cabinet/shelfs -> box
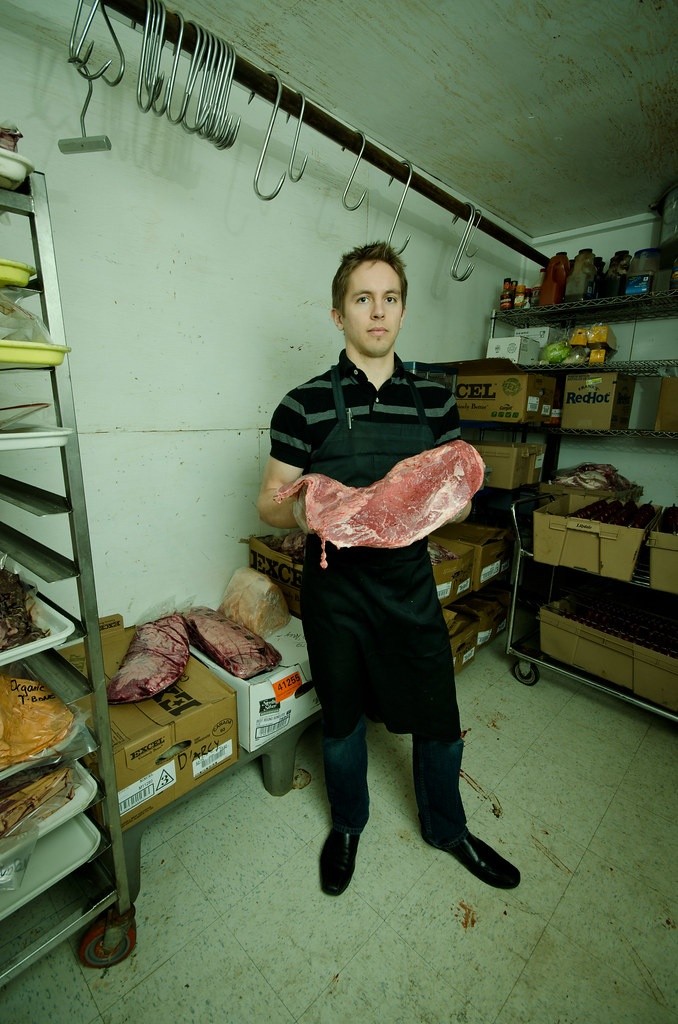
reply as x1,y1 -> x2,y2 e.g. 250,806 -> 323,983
0,170 -> 139,987
491,288 -> 678,439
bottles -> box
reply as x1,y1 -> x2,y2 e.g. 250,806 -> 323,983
500,248 -> 658,310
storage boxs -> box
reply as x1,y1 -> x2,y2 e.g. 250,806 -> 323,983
536,597 -> 633,691
560,371 -> 637,430
427,356 -> 557,423
247,525 -> 515,674
189,617 -> 322,753
514,326 -> 561,349
569,324 -> 618,364
490,440 -> 546,484
469,440 -> 531,490
632,642 -> 678,711
486,336 -> 540,365
655,376 -> 678,432
644,506 -> 678,594
56,614 -> 239,832
532,493 -> 663,582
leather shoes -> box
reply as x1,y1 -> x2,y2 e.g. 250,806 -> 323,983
419,818 -> 521,889
319,827 -> 360,895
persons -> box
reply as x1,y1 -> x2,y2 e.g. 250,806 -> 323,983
257,242 -> 522,896
